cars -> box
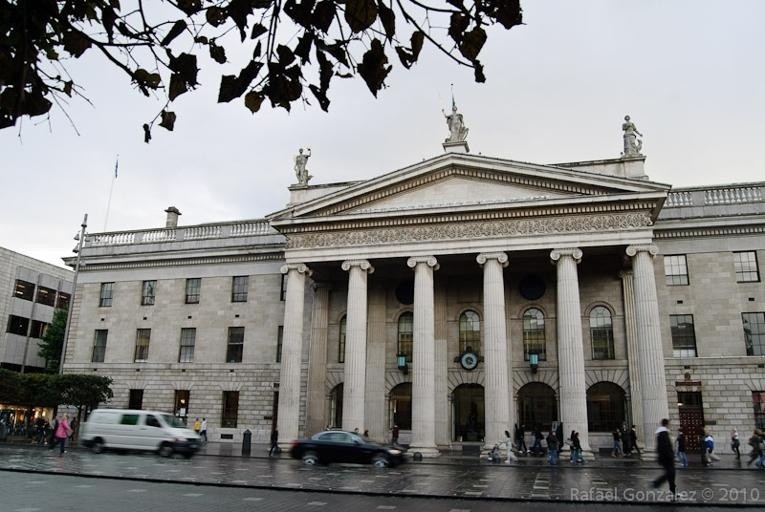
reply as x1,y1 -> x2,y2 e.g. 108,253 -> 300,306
288,429 -> 404,471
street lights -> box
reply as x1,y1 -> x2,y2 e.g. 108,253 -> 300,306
56,213 -> 91,377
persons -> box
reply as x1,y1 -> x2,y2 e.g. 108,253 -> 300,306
676,427 -> 689,465
69,415 -> 76,439
745,429 -> 762,464
390,424 -> 400,442
268,426 -> 279,457
194,415 -> 200,432
324,424 -> 331,430
705,432 -> 721,463
354,427 -> 359,432
699,427 -> 706,463
650,417 -> 681,502
50,411 -> 73,453
50,414 -> 58,430
293,147 -> 311,184
363,429 -> 369,436
199,417 -> 207,441
755,431 -> 765,467
731,426 -> 741,458
622,115 -> 643,150
499,419 -> 643,463
440,106 -> 465,142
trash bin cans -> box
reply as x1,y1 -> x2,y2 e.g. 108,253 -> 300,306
243,429 -> 252,445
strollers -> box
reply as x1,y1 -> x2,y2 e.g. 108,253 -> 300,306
487,442 -> 503,462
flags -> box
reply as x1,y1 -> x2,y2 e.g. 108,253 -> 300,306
114,161 -> 118,178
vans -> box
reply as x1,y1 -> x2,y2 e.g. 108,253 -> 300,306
82,407 -> 203,459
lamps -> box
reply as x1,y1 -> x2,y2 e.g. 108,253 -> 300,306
396,351 -> 408,374
528,349 -> 539,373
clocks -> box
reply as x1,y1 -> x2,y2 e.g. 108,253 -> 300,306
460,351 -> 478,369
